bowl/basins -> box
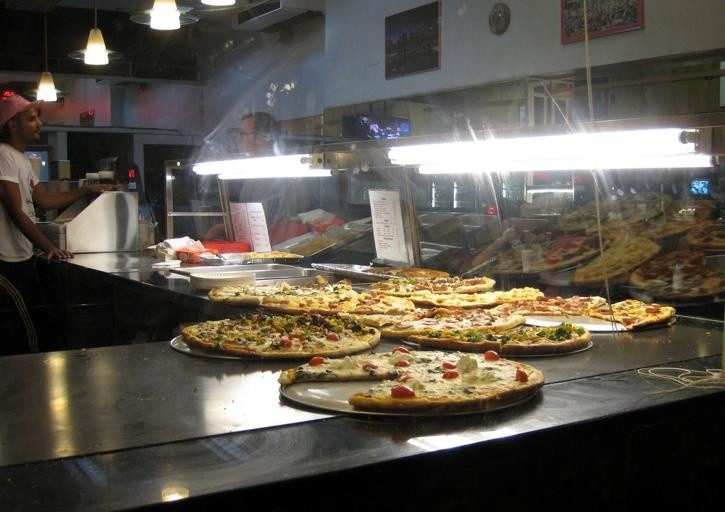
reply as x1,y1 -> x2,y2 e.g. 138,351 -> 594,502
189,271 -> 252,292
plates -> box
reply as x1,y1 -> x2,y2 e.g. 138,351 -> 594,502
168,335 -> 256,363
250,271 -> 335,287
276,371 -> 542,419
398,336 -> 596,359
630,253 -> 725,308
311,263 -> 449,282
171,263 -> 302,272
523,314 -> 634,335
543,260 -> 585,277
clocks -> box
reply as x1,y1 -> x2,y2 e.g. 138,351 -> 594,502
488,3 -> 511,35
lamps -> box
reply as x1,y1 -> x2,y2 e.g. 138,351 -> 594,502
191,155 -> 312,176
412,152 -> 725,175
217,167 -> 338,180
84,28 -> 111,66
149,0 -> 182,31
384,126 -> 703,167
36,71 -> 58,103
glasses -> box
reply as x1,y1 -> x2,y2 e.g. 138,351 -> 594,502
236,132 -> 253,140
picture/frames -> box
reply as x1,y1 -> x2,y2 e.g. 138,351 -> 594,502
559,0 -> 645,46
384,1 -> 442,81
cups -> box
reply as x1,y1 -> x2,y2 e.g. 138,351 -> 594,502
522,248 -> 534,276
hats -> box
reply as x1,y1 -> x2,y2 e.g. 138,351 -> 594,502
0,94 -> 45,127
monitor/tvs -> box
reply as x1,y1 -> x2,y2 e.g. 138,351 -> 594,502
357,115 -> 410,139
21,145 -> 50,180
688,180 -> 709,196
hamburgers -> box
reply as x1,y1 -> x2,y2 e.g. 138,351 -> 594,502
639,199 -> 712,237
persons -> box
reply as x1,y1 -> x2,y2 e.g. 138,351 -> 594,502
2,92 -> 75,297
199,108 -> 297,242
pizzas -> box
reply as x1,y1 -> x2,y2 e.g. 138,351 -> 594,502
403,286 -> 545,308
257,290 -> 415,315
473,228 -> 610,277
688,218 -> 725,247
519,294 -> 608,316
407,325 -> 590,354
277,347 -> 544,413
182,314 -> 383,355
364,266 -> 449,279
367,276 -> 496,296
572,234 -> 662,284
591,297 -> 677,329
559,189 -> 672,227
630,249 -> 725,300
382,304 -> 527,337
207,275 -> 353,306
